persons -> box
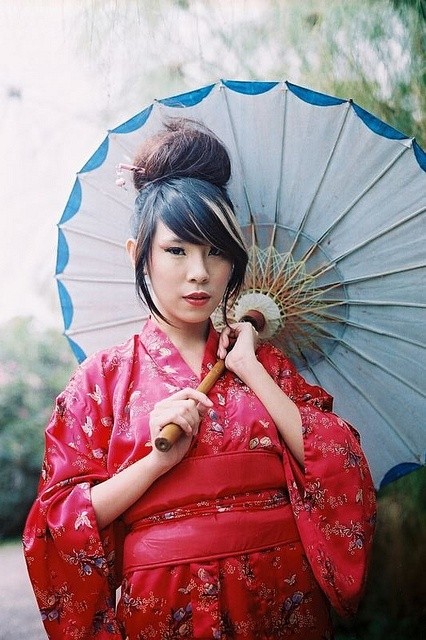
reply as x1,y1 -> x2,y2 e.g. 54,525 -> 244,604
22,116 -> 378,640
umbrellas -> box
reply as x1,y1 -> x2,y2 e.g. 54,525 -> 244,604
53,79 -> 426,492
